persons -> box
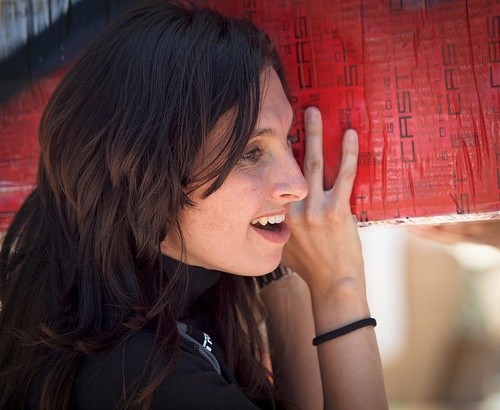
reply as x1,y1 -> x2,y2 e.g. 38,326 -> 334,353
0,0 -> 389,410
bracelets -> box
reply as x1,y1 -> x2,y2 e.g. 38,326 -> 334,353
255,264 -> 296,289
312,318 -> 376,346
